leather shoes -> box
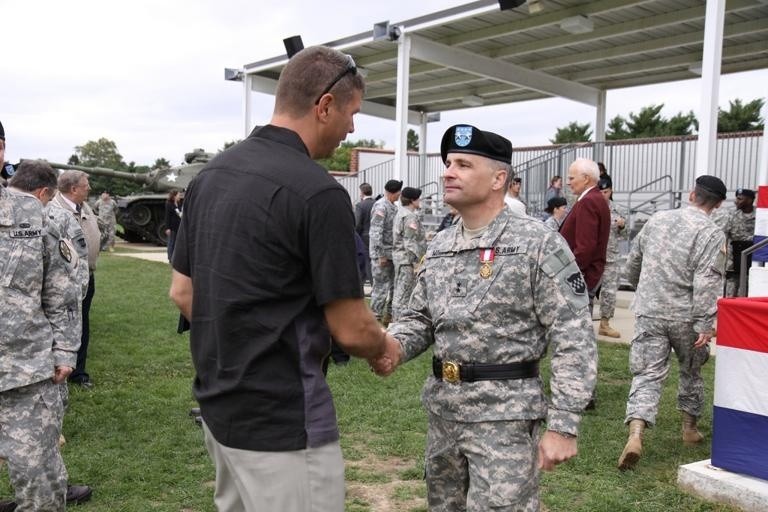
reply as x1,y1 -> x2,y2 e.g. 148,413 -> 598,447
65,485 -> 92,503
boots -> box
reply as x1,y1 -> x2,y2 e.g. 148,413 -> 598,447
680,409 -> 705,443
595,316 -> 624,339
617,415 -> 647,472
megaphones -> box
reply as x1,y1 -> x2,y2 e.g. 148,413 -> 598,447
427,112 -> 440,122
225,68 -> 243,81
374,21 -> 392,42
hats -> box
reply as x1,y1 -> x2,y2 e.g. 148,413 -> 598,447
384,179 -> 403,193
599,178 -> 611,190
735,189 -> 755,198
441,124 -> 513,164
547,197 -> 567,208
695,175 -> 726,198
401,187 -> 422,199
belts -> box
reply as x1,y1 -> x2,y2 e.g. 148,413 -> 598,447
429,355 -> 541,384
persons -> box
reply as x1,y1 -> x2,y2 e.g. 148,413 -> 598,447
164,187 -> 187,262
1,159 -> 101,512
93,190 -> 120,252
370,124 -> 597,512
1,122 -> 67,512
708,189 -> 757,337
353,162 -> 626,338
168,45 -> 403,511
618,175 -> 728,471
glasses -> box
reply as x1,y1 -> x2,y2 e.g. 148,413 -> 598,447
314,49 -> 359,108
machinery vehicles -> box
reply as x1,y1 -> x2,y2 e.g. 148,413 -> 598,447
19,148 -> 222,247
